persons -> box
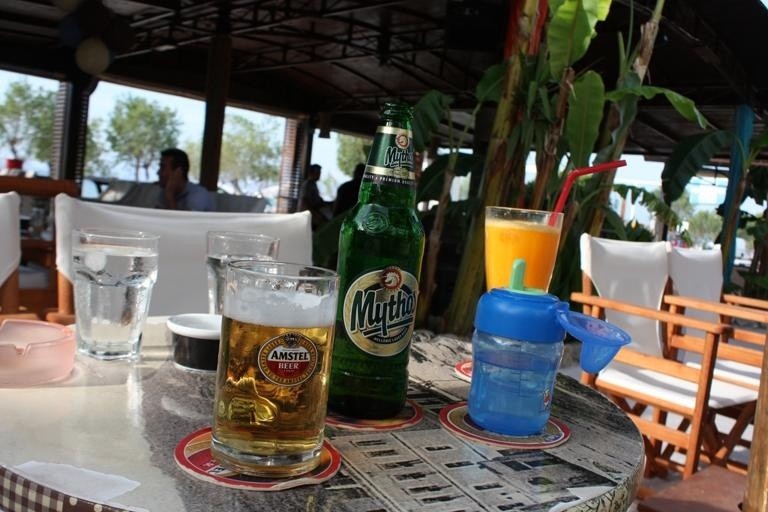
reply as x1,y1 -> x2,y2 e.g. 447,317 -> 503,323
143,149 -> 215,212
295,164 -> 331,230
332,162 -> 366,216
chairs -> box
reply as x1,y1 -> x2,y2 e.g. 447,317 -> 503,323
2,187 -> 27,316
654,238 -> 767,479
568,229 -> 767,484
113,181 -> 267,213
48,194 -> 322,319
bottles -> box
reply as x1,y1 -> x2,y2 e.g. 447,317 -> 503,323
470,282 -> 632,436
327,96 -> 427,420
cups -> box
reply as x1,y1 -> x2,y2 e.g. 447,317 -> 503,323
205,229 -> 282,316
71,226 -> 163,360
212,259 -> 339,479
483,203 -> 563,291
20,216 -> 31,230
168,313 -> 224,377
31,200 -> 48,228
160,382 -> 213,417
